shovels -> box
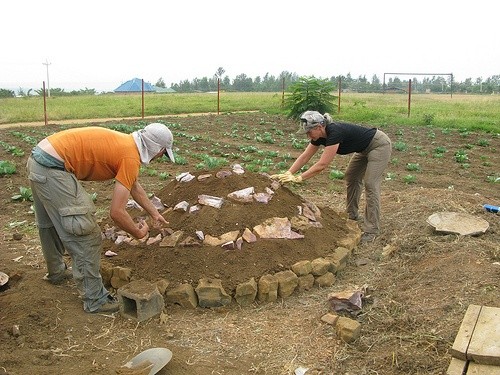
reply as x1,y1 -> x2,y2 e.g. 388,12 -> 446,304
116,347 -> 173,375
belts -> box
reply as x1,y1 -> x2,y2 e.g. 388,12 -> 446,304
29,152 -> 68,171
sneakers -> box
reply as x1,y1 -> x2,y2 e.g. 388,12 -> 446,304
93,294 -> 121,313
51,263 -> 73,283
361,232 -> 376,242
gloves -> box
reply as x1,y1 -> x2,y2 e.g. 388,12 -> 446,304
269,171 -> 303,185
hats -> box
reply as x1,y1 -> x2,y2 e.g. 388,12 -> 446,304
295,110 -> 324,135
131,123 -> 175,165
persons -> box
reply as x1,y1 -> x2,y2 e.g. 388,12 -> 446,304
269,110 -> 392,243
25,122 -> 176,313
